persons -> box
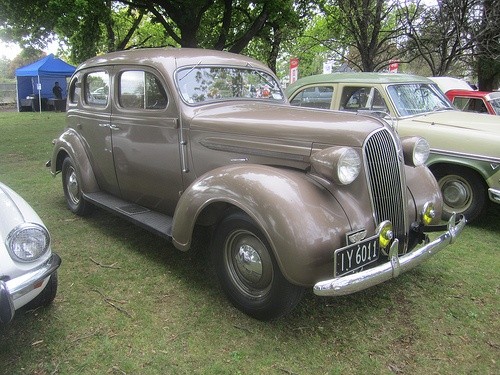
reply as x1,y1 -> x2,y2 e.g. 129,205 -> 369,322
53,82 -> 63,99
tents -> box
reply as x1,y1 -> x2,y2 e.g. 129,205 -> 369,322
15,53 -> 77,113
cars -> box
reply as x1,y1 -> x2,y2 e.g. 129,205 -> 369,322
0,181 -> 62,328
47,46 -> 467,322
445,89 -> 500,116
286,73 -> 500,225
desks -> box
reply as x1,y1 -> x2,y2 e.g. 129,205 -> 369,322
26,98 -> 38,112
43,97 -> 61,113
